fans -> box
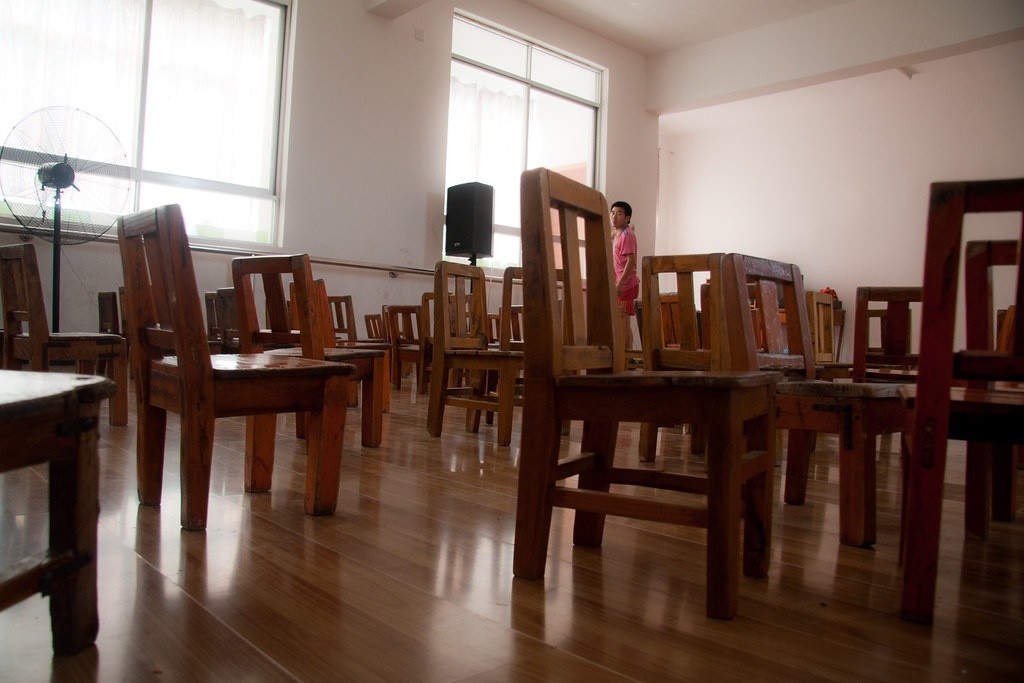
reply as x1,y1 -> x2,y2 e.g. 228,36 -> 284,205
0,105 -> 131,334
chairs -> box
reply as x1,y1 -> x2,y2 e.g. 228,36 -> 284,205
0,166 -> 1024,683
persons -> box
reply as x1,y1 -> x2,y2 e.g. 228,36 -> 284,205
610,201 -> 641,372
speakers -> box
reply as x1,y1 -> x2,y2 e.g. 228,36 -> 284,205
446,182 -> 493,260
